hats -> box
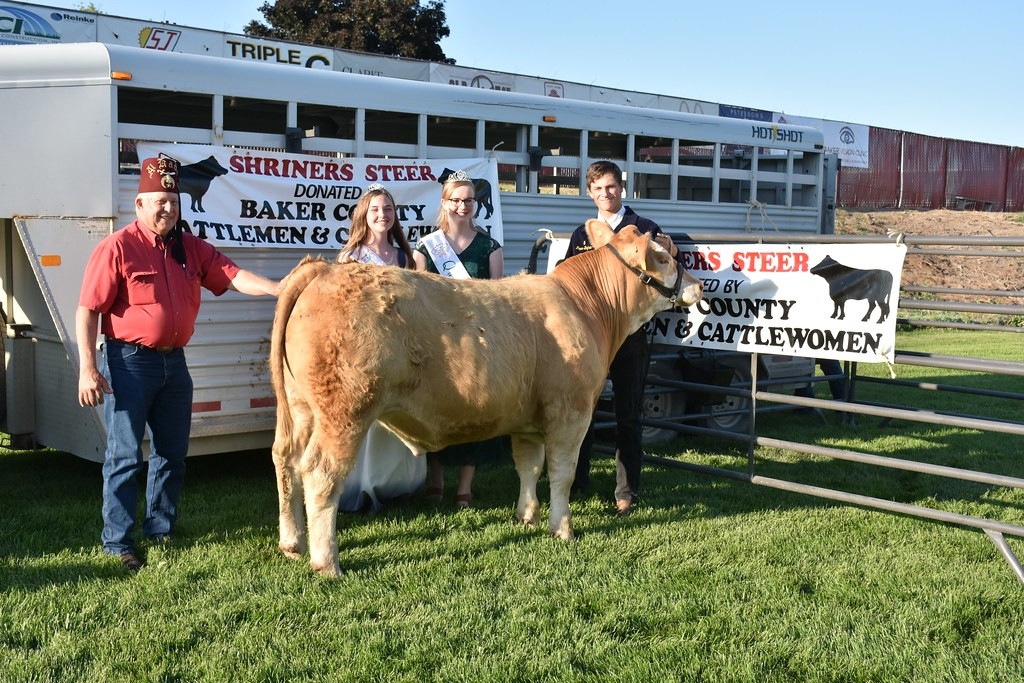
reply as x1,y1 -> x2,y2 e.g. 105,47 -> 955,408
137,158 -> 180,196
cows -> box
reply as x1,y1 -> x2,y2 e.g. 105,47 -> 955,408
267,219 -> 706,579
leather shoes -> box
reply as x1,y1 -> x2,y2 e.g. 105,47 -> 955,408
569,479 -> 591,497
149,533 -> 173,541
116,553 -> 141,569
616,498 -> 632,516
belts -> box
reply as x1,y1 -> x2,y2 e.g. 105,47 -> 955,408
103,332 -> 178,354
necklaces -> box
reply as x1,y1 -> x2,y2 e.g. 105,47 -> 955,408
384,254 -> 394,265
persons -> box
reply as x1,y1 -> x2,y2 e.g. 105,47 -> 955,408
337,184 -> 427,517
792,358 -> 857,423
556,160 -> 687,515
409,169 -> 503,512
75,158 -> 291,572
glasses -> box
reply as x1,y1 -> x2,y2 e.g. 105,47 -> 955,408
446,197 -> 477,207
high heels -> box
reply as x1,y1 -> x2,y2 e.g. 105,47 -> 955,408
429,479 -> 445,501
457,484 -> 473,508
